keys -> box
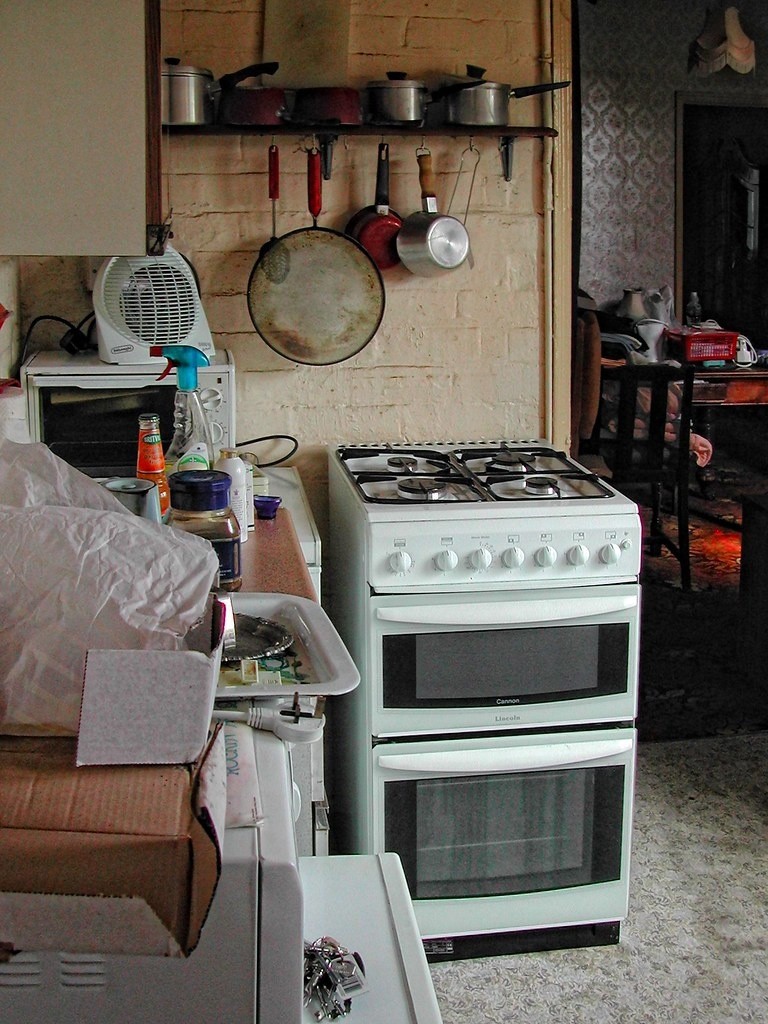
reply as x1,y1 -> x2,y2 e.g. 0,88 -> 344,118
304,936 -> 372,1022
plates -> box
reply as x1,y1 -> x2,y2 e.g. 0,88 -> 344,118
221,614 -> 294,661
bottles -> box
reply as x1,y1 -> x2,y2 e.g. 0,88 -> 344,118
686,292 -> 701,327
163,471 -> 243,593
136,414 -> 170,524
212,448 -> 248,543
238,454 -> 255,531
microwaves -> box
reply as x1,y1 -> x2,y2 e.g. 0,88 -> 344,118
21,350 -> 235,478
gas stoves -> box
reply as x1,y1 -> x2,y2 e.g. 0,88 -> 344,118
328,438 -> 642,593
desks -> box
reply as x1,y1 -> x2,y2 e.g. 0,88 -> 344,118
675,351 -> 768,501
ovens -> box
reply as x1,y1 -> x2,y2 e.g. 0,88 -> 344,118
355,586 -> 642,964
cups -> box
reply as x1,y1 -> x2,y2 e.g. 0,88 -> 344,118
255,495 -> 282,519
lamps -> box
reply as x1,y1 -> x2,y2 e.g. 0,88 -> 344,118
687,6 -> 756,80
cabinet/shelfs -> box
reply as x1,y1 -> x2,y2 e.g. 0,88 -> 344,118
0,0 -> 165,256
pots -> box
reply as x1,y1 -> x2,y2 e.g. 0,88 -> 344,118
397,147 -> 469,277
367,72 -> 487,121
344,143 -> 405,270
160,57 -> 279,127
444,64 -> 571,126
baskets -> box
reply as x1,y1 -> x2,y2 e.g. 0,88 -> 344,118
664,328 -> 740,361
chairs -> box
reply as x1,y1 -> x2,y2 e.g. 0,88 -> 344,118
573,363 -> 696,589
